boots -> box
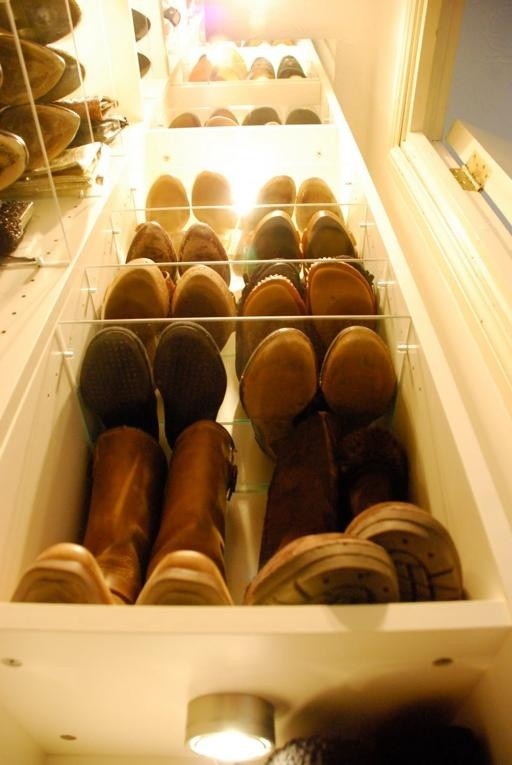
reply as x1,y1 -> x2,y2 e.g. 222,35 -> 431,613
10,410 -> 460,605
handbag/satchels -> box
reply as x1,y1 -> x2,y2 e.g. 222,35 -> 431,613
0,200 -> 34,256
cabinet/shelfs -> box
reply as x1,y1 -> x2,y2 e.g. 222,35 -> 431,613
0,0 -> 512,765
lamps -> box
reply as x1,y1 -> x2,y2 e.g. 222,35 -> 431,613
185,692 -> 275,763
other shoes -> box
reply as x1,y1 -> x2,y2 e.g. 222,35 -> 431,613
81,172 -> 397,452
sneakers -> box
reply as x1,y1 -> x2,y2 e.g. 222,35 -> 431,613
172,49 -> 320,127
0,0 -> 126,191
